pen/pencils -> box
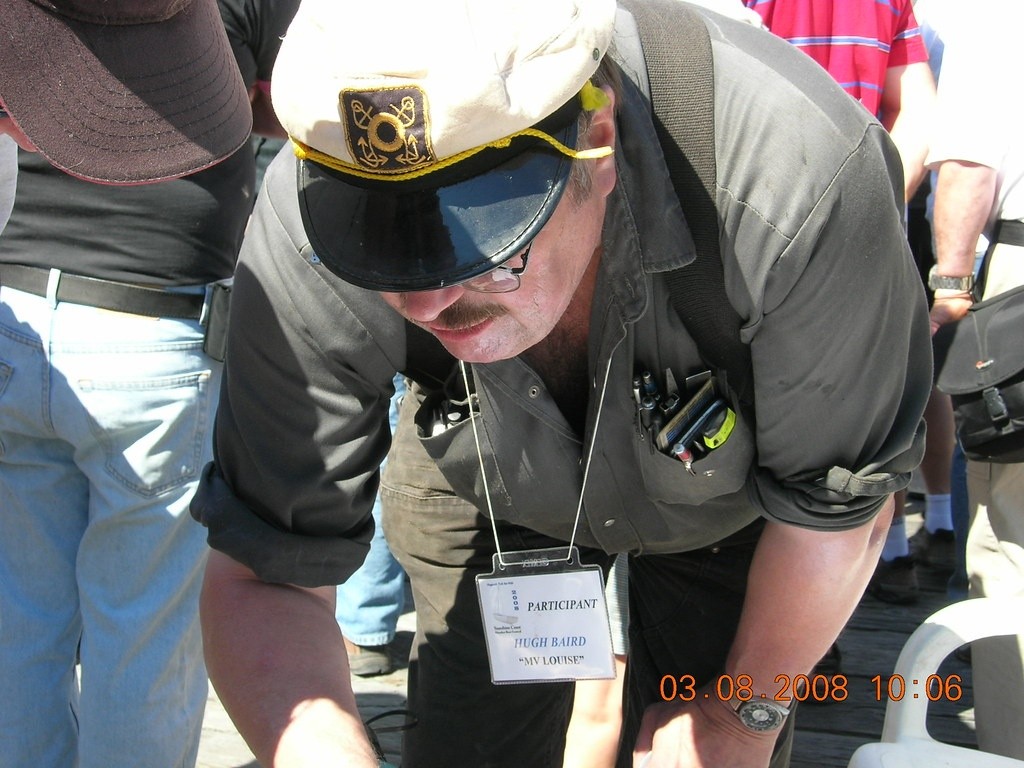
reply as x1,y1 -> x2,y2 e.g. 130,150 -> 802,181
671,443 -> 698,478
633,371 -> 664,455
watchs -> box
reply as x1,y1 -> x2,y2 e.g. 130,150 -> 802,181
714,674 -> 790,731
928,264 -> 975,291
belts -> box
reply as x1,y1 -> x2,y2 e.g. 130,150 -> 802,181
0,262 -> 205,320
992,220 -> 1024,247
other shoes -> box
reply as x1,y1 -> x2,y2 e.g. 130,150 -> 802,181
345,635 -> 392,675
907,526 -> 957,593
868,554 -> 920,602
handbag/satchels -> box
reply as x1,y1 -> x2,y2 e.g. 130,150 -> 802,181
933,285 -> 1024,464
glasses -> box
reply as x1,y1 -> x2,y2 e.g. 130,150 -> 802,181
455,239 -> 532,294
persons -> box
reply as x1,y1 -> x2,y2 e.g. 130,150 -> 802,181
190,0 -> 934,768
0,1 -> 1024,768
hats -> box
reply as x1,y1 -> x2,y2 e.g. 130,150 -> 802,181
0,0 -> 253,185
270,0 -> 609,293
